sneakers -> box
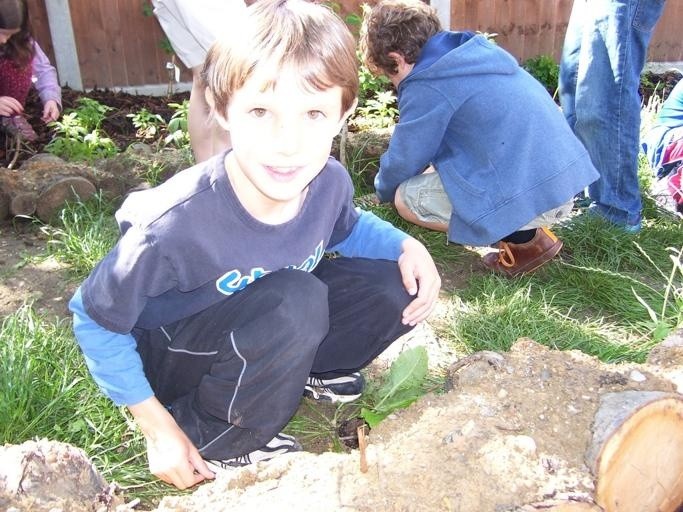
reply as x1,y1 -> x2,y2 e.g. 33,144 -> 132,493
478,225 -> 565,280
302,371 -> 367,404
192,431 -> 304,479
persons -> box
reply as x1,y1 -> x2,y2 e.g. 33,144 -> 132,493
67,0 -> 444,495
1,0 -> 64,143
641,76 -> 682,210
558,0 -> 667,235
151,0 -> 249,166
358,0 -> 600,278
